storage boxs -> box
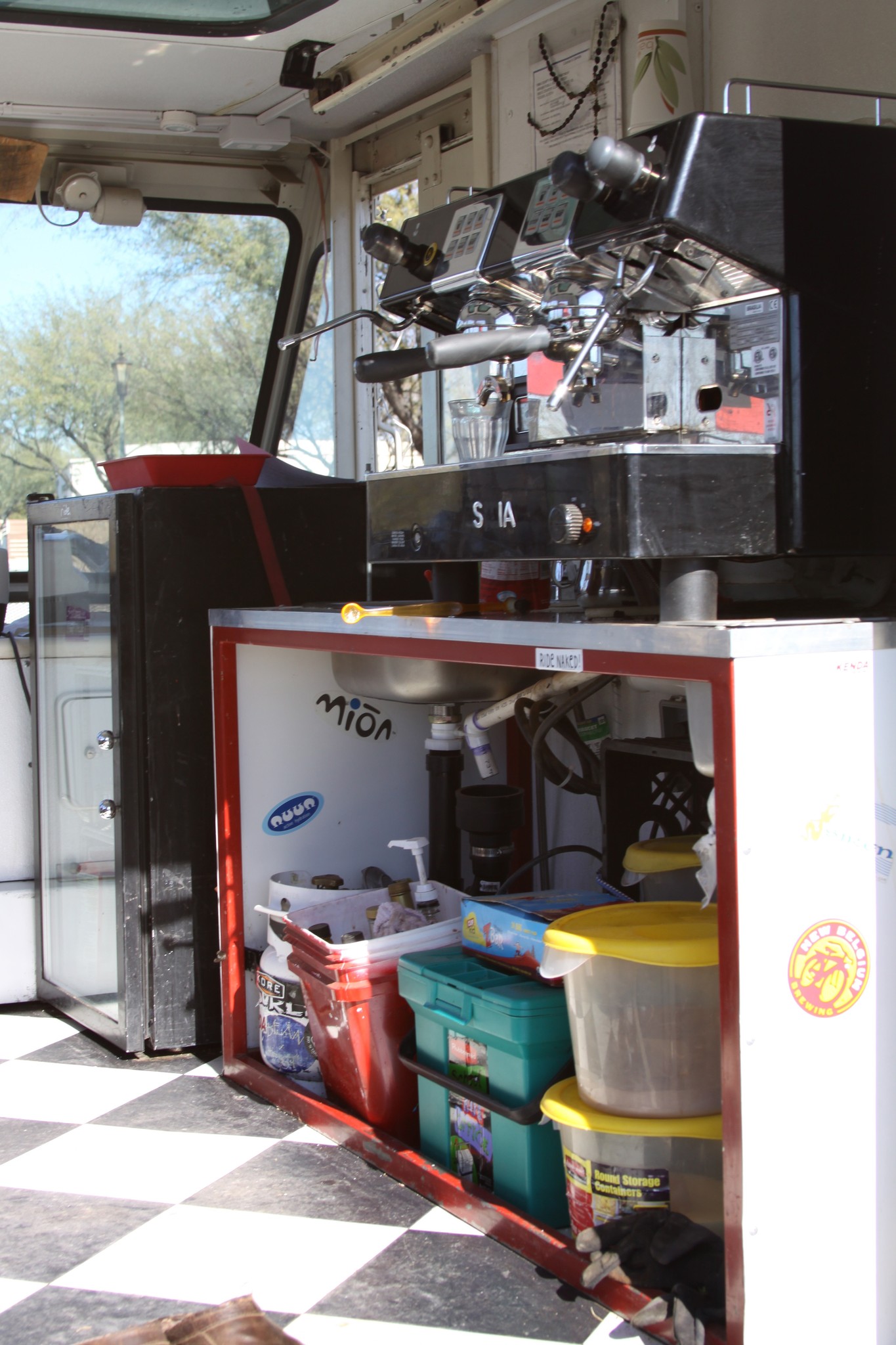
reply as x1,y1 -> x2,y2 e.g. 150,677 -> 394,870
282,737 -> 717,1228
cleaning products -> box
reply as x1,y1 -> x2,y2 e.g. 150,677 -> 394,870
388,836 -> 443,921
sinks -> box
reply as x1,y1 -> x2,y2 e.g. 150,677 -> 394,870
330,605 -> 528,706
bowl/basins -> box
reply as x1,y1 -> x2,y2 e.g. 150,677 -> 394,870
537,1076 -> 726,1242
622,834 -> 707,904
536,902 -> 724,1119
97,454 -> 273,490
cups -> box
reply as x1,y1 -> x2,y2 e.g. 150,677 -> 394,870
448,397 -> 513,462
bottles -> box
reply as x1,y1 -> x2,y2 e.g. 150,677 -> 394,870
309,881 -> 416,943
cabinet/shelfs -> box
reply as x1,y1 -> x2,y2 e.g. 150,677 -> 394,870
211,605 -> 896,1345
